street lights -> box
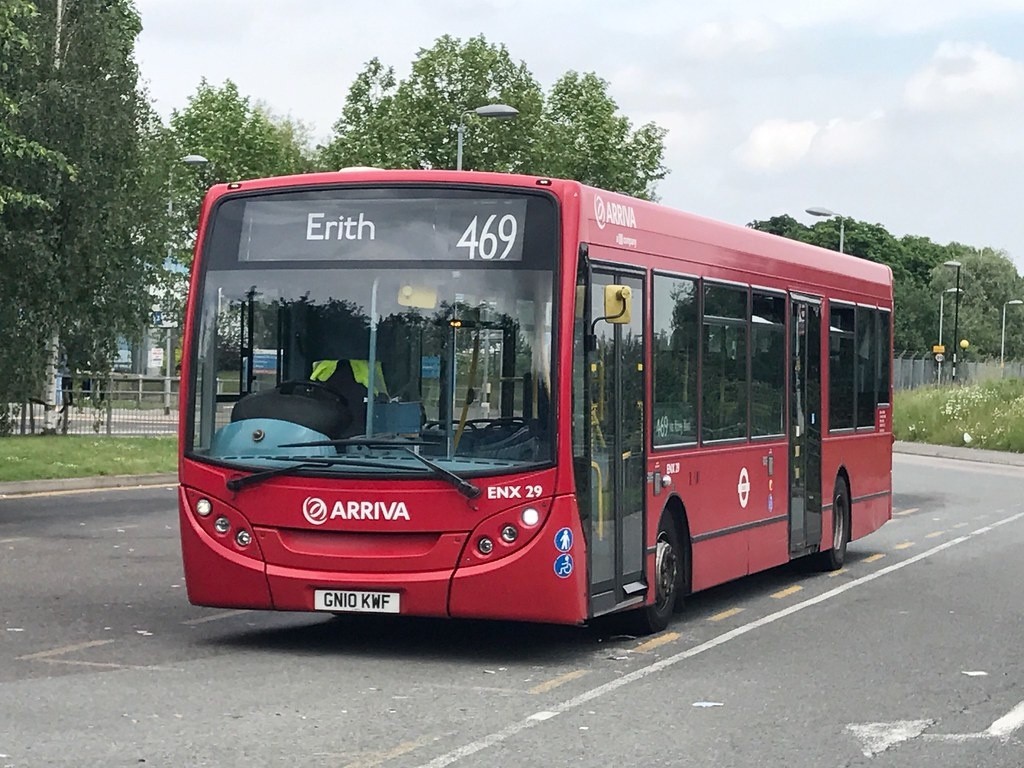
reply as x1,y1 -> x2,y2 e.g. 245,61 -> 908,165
456,104 -> 520,172
943,260 -> 957,386
936,287 -> 963,385
960,340 -> 969,386
805,206 -> 845,253
1000,300 -> 1023,389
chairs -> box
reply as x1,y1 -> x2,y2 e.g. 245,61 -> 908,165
312,357 -> 385,437
428,418 -> 538,458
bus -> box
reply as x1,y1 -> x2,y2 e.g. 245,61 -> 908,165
178,166 -> 895,634
